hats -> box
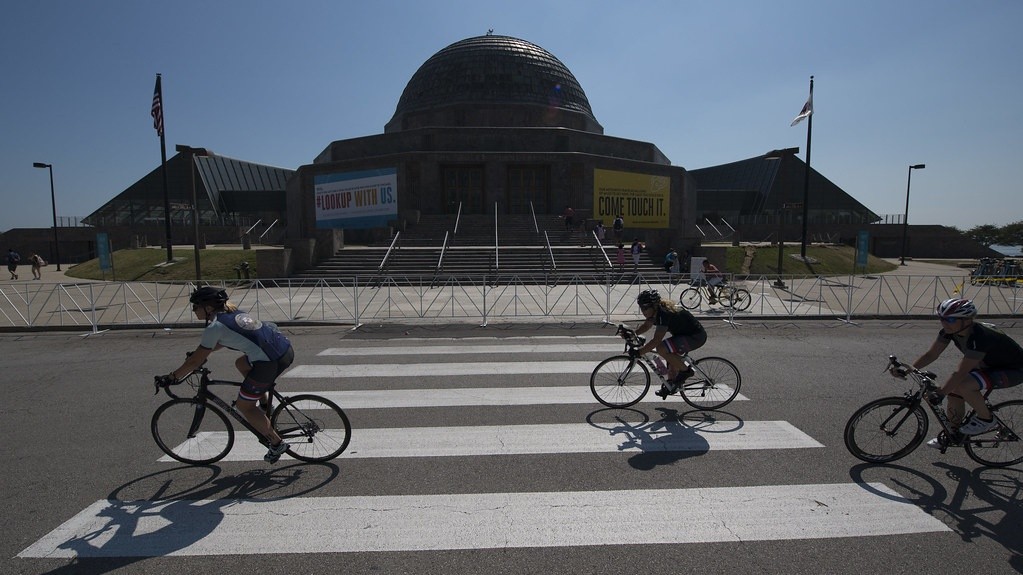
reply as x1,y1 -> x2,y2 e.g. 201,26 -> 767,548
673,252 -> 677,256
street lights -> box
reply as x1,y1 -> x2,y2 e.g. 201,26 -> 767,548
33,162 -> 63,271
764,146 -> 799,286
901,164 -> 925,264
175,143 -> 216,290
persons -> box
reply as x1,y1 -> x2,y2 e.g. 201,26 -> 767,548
27,249 -> 42,281
613,214 -> 625,245
700,260 -> 723,304
630,237 -> 643,268
5,248 -> 20,280
618,290 -> 707,398
888,298 -> 1023,448
563,204 -> 576,231
616,245 -> 626,270
664,247 -> 693,284
596,222 -> 606,246
154,287 -> 294,462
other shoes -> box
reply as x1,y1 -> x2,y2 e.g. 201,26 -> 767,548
655,382 -> 677,395
673,367 -> 694,384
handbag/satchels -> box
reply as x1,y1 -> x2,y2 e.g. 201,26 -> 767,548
691,278 -> 707,287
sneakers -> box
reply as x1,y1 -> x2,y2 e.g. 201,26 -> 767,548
959,415 -> 998,434
927,434 -> 950,448
264,441 -> 290,461
256,404 -> 275,415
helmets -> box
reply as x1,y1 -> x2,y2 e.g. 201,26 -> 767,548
190,287 -> 229,303
936,299 -> 976,317
638,289 -> 661,306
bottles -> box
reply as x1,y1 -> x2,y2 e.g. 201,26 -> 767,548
652,354 -> 667,375
231,400 -> 248,422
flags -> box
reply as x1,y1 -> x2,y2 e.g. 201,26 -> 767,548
151,79 -> 162,137
790,84 -> 815,126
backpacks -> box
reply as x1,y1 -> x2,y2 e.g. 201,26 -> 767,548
614,218 -> 621,230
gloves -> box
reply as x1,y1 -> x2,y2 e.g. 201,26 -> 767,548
929,389 -> 944,405
154,372 -> 178,388
890,367 -> 907,376
628,347 -> 640,356
621,330 -> 634,338
185,351 -> 196,360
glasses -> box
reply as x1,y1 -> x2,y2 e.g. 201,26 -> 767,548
939,317 -> 967,322
193,304 -> 206,310
641,306 -> 653,312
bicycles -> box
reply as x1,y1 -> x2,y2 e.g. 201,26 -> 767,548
589,320 -> 742,411
151,350 -> 352,465
970,256 -> 1014,287
843,354 -> 1023,468
680,269 -> 752,312
